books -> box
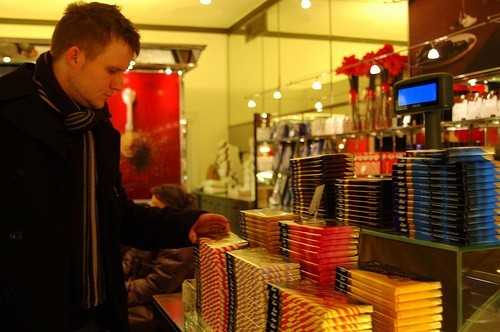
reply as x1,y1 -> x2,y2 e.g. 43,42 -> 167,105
193,207 -> 444,332
290,147 -> 500,247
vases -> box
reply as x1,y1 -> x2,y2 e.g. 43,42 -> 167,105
349,90 -> 390,130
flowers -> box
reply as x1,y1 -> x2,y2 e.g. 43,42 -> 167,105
335,43 -> 408,86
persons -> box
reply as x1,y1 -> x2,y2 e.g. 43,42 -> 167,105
123,184 -> 197,332
0,1 -> 230,332
